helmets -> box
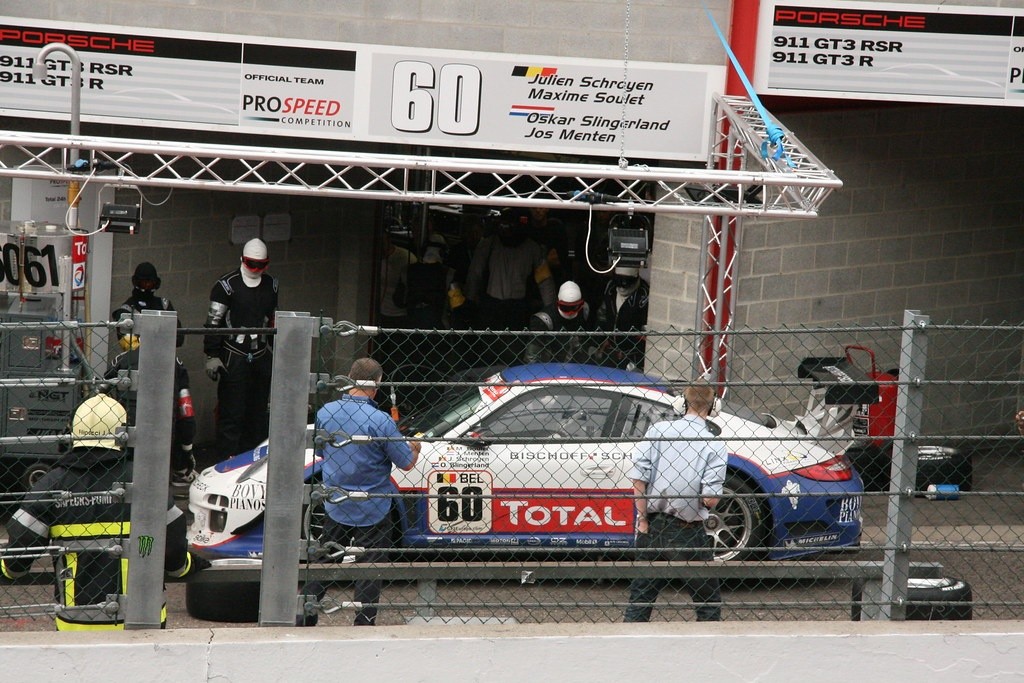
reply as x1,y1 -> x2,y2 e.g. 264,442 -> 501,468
131,262 -> 161,290
242,238 -> 267,278
616,267 -> 639,295
70,393 -> 127,451
495,208 -> 521,242
558,281 -> 582,319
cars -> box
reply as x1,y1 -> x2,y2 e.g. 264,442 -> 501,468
186,363 -> 863,561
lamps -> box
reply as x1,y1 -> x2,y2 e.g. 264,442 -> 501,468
607,214 -> 651,266
687,183 -> 763,207
96,184 -> 144,233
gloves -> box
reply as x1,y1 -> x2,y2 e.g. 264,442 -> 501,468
177,552 -> 212,580
205,357 -> 228,381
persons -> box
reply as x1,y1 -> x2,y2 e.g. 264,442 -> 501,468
410,215 -> 454,267
529,279 -> 593,365
203,235 -> 293,465
623,379 -> 729,622
442,214 -> 499,285
595,261 -> 651,372
375,223 -> 419,418
293,358 -> 420,627
464,214 -> 560,309
0,394 -> 212,630
111,261 -> 185,354
103,335 -> 198,483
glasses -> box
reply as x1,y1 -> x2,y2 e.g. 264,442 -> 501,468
135,279 -> 156,289
243,257 -> 269,272
615,274 -> 638,288
496,221 -> 514,232
558,300 -> 584,316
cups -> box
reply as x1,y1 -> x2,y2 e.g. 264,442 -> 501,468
927,484 -> 959,501
